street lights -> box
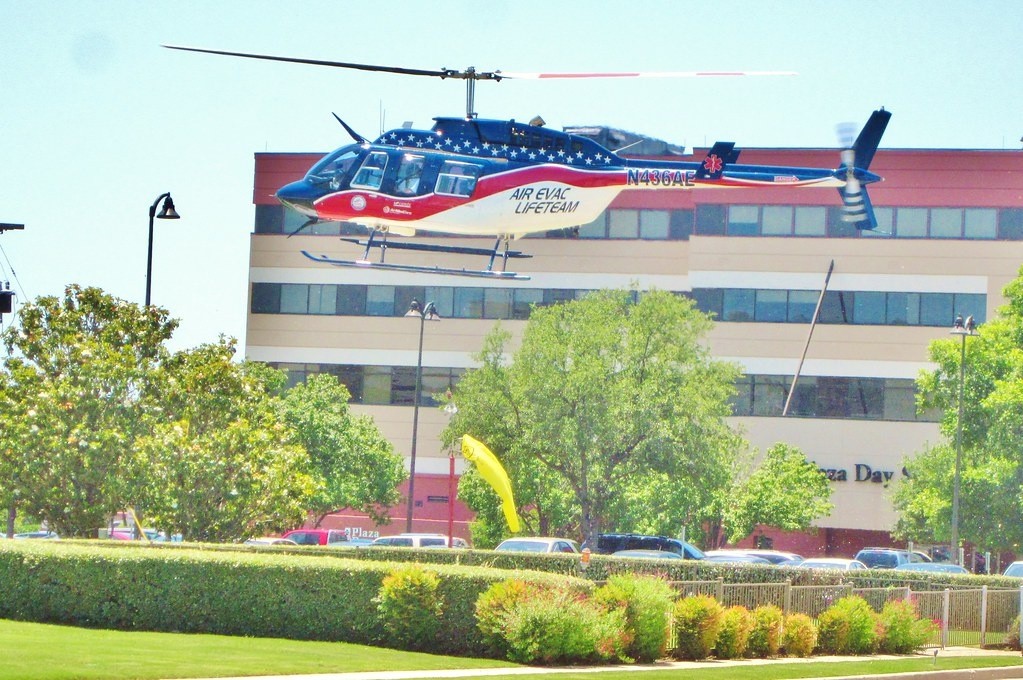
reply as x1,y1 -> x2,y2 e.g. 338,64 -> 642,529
950,311 -> 981,564
403,297 -> 441,532
144,192 -> 180,309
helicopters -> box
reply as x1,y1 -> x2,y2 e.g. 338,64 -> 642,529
159,42 -> 893,281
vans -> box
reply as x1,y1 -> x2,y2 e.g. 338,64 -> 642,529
855,547 -> 932,568
370,532 -> 468,548
595,532 -> 705,560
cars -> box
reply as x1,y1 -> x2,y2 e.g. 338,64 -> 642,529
11,528 -> 346,546
1003,560 -> 1022,576
610,549 -> 970,577
491,537 -> 584,553
